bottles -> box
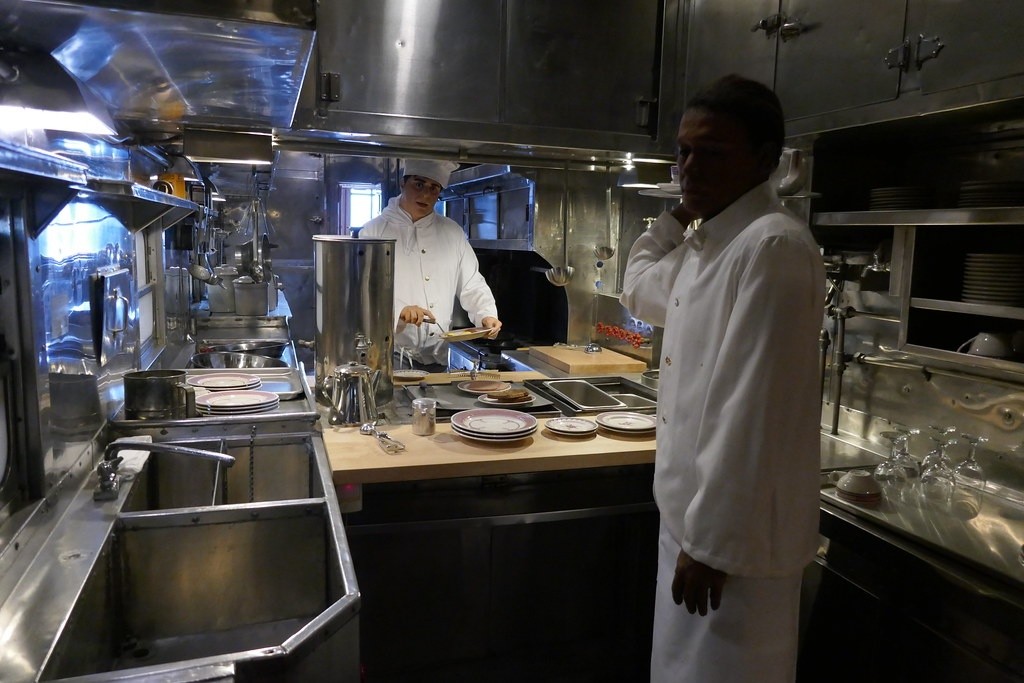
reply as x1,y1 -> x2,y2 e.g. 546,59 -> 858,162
265,260 -> 278,312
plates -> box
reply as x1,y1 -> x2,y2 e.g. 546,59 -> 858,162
438,326 -> 492,342
867,186 -> 936,210
478,394 -> 536,405
392,370 -> 429,378
961,253 -> 1022,306
451,409 -> 538,442
183,374 -> 262,391
956,182 -> 1024,207
457,381 -> 511,394
195,390 -> 280,417
544,418 -> 597,436
595,412 -> 655,434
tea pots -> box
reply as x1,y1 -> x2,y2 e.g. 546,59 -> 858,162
322,361 -> 381,427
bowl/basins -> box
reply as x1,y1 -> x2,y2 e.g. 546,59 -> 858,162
839,250 -> 901,314
232,276 -> 254,284
198,341 -> 289,359
190,353 -> 290,367
836,470 -> 883,508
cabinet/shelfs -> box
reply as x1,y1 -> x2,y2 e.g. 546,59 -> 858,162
265,0 -> 1024,380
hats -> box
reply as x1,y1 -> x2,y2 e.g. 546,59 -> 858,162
403,157 -> 461,190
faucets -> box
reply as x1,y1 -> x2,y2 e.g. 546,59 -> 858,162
861,244 -> 892,278
92,439 -> 236,500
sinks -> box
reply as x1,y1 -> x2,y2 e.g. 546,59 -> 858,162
120,441 -> 221,512
214,436 -> 325,505
37,505 -> 348,680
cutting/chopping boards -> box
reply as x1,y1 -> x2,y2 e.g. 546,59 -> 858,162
529,345 -> 647,374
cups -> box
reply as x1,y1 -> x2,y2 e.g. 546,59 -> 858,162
956,332 -> 1024,357
233,282 -> 268,315
122,370 -> 195,421
209,276 -> 238,313
412,398 -> 436,436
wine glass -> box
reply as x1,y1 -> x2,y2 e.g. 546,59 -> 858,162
877,424 -> 989,500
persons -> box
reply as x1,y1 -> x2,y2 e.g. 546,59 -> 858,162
358,158 -> 502,371
622,76 -> 825,683
